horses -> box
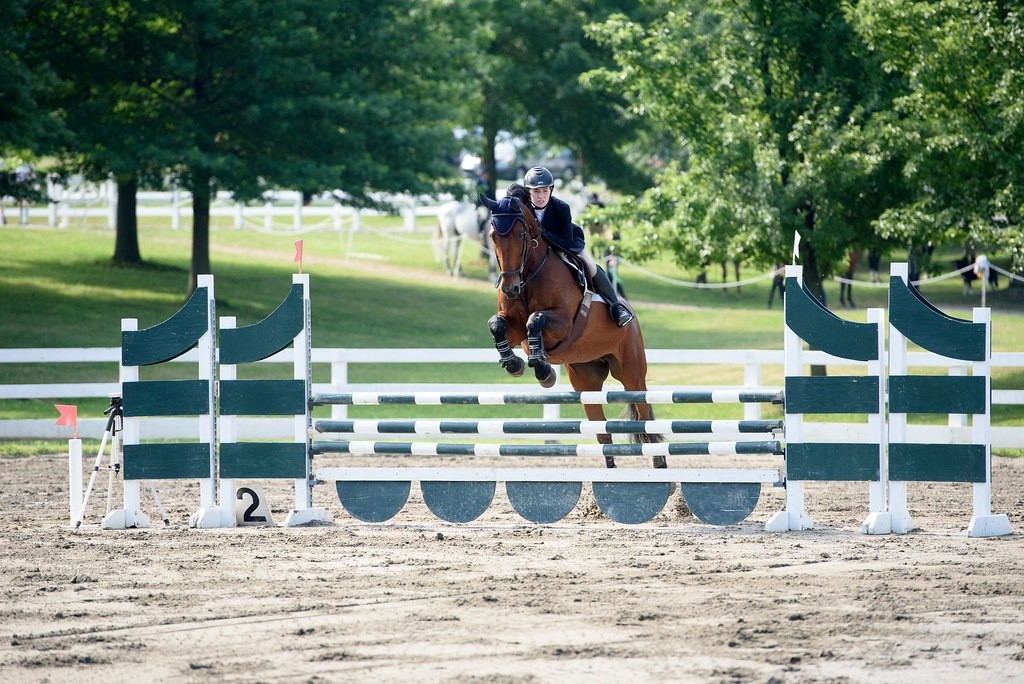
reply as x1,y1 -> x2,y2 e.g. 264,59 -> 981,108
479,192 -> 670,469
435,200 -> 501,285
766,258 -> 1000,310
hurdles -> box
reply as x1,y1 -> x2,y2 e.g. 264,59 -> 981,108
96,258 -> 1017,541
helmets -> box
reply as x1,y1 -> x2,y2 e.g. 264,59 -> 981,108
524,167 -> 555,188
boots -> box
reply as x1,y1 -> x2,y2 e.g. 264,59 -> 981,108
592,264 -> 630,327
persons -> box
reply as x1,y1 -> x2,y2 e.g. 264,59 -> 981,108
474,169 -> 494,235
690,250 -> 881,310
523,166 -> 633,328
